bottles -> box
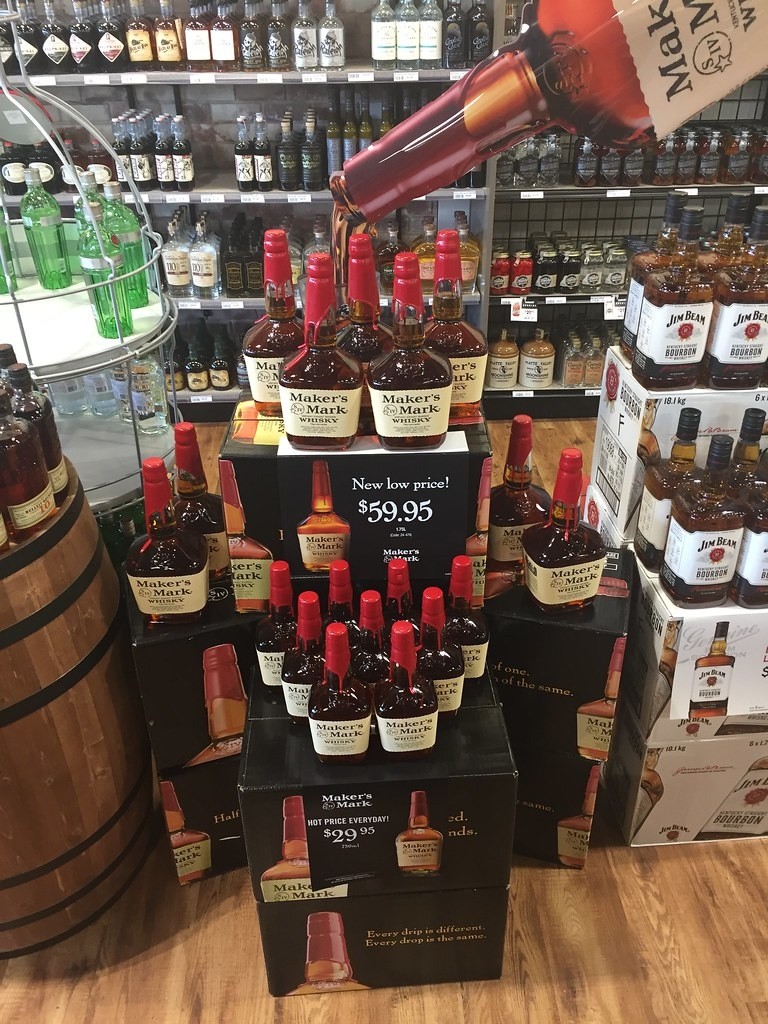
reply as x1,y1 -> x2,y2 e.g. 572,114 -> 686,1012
393,791 -> 443,874
219,459 -> 273,610
480,559 -> 630,599
0,1 -> 766,756
631,768 -> 665,845
260,796 -> 347,901
328,0 -> 768,229
285,912 -> 370,997
576,638 -> 626,761
296,459 -> 350,573
230,398 -> 286,448
160,781 -> 211,886
466,457 -> 492,609
692,756 -> 768,841
179,643 -> 247,767
624,398 -> 661,534
688,622 -> 735,720
647,649 -> 677,740
556,766 -> 602,869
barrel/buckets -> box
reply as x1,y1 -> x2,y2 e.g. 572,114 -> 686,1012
0,456 -> 155,965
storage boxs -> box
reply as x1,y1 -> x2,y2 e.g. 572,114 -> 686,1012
124,346 -> 768,997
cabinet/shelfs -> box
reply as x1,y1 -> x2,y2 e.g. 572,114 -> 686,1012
0,0 -> 768,515
